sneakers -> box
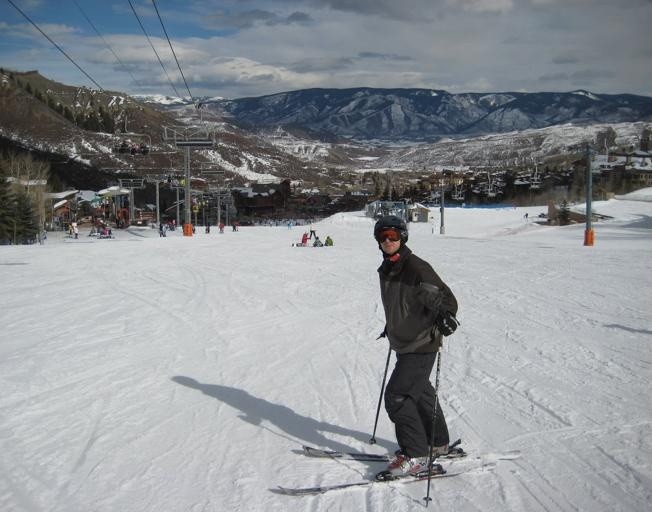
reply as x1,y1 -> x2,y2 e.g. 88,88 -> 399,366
388,442 -> 449,478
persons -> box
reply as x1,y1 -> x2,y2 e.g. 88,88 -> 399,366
158,194 -> 241,238
119,140 -> 150,156
55,213 -> 79,239
94,209 -> 121,239
374,214 -> 460,476
259,214 -> 312,230
301,232 -> 333,248
309,223 -> 316,239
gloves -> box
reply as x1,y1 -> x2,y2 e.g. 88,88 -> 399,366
436,310 -> 460,336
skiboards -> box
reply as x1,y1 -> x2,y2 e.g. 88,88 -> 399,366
277,445 -> 523,494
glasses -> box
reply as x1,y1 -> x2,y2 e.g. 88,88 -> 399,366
374,226 -> 409,242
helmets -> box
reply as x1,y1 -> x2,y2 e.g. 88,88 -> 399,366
374,215 -> 408,243
